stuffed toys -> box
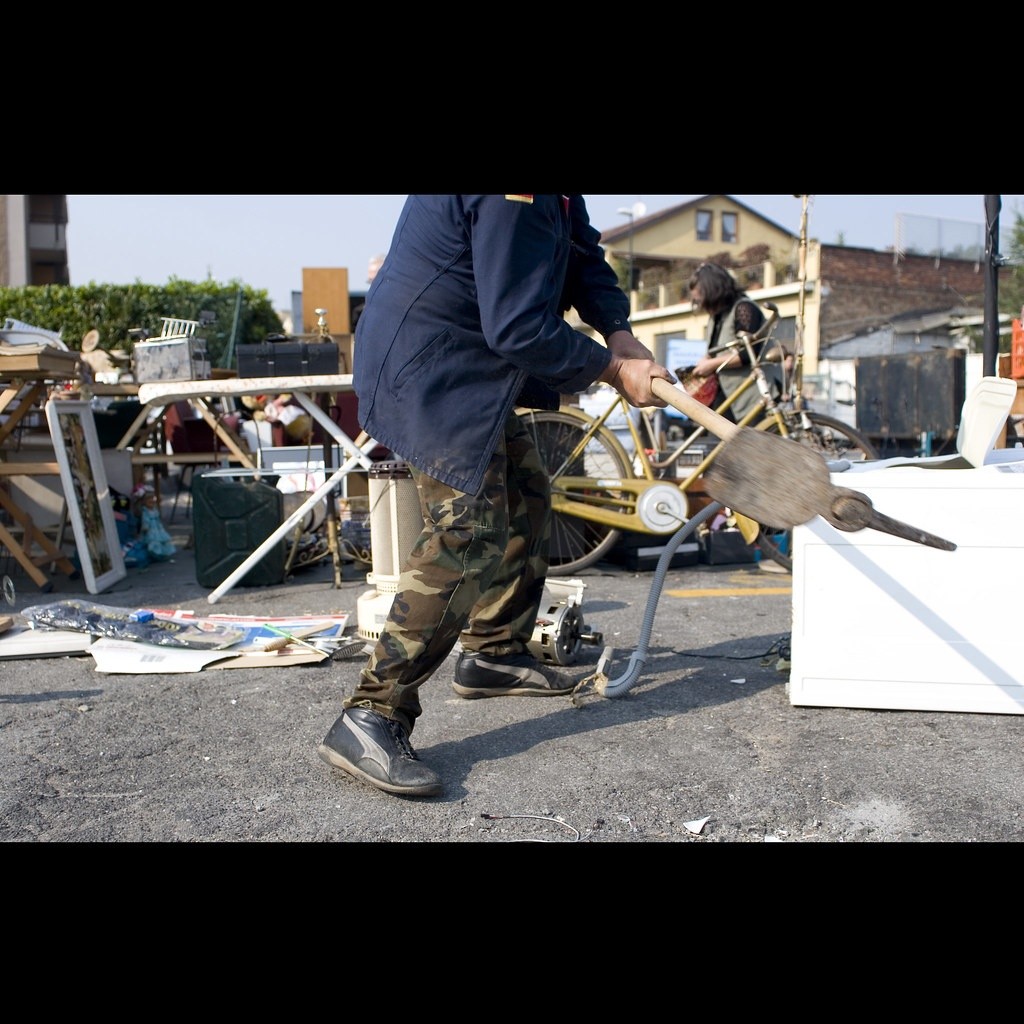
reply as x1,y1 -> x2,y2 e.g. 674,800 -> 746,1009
244,394 -> 315,442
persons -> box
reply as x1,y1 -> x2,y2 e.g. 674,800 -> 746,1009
318,193 -> 677,799
785,351 -> 794,392
686,261 -> 783,533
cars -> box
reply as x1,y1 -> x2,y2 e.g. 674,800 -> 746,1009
578,337 -> 712,570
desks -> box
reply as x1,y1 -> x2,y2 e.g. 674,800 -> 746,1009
75,380 -> 258,482
138,373 -> 383,604
851,376 -> 1024,728
0,345 -> 83,592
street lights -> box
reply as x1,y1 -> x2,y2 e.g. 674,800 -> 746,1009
616,207 -> 634,292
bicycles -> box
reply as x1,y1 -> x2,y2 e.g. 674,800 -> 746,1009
512,298 -> 880,577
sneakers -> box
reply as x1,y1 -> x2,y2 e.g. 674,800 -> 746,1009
452,642 -> 577,699
317,707 -> 444,795
